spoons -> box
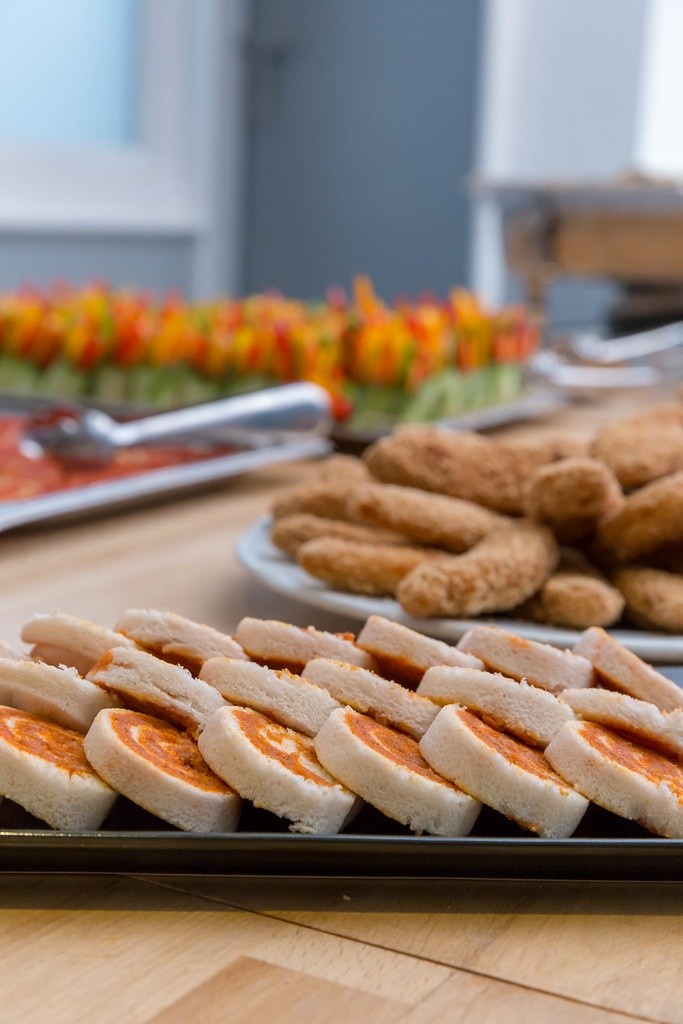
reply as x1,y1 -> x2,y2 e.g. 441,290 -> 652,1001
18,381 -> 332,475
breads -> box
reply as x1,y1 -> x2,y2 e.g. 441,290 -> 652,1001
267,401 -> 683,633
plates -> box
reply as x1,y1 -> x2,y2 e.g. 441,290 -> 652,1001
1,800 -> 683,858
0,396 -> 328,535
236,513 -> 683,667
333,395 -> 570,443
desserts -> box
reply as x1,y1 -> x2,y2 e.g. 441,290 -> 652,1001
0,606 -> 683,839
